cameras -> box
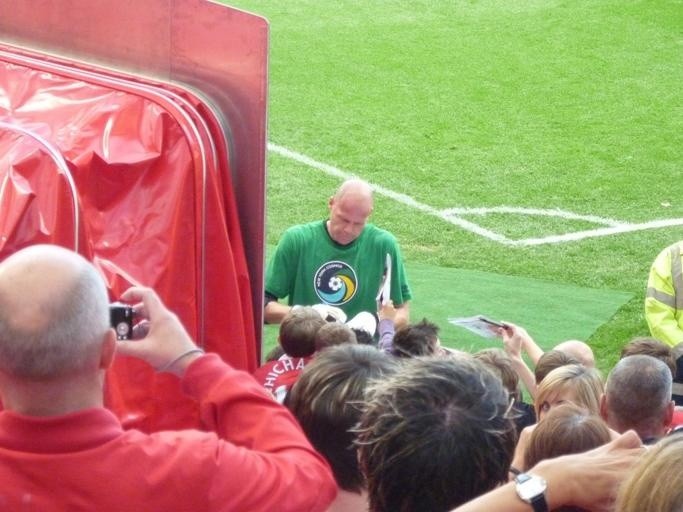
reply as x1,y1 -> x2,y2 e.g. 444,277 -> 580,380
108,304 -> 133,343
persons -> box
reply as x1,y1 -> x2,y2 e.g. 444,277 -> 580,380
0,243 -> 339,512
264,178 -> 412,346
644,241 -> 682,356
248,303 -> 682,511
